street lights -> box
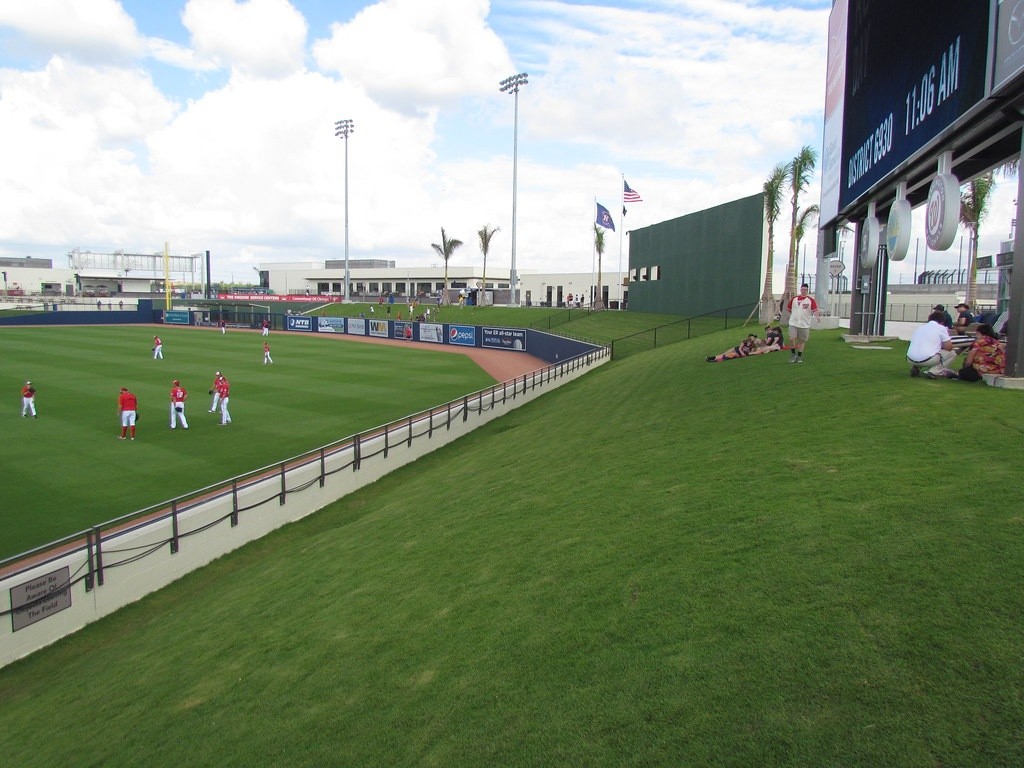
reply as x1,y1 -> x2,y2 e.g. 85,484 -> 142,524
500,73 -> 529,308
335,119 -> 355,305
836,240 -> 846,293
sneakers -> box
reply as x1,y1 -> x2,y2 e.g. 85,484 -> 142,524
797,356 -> 802,363
789,353 -> 797,362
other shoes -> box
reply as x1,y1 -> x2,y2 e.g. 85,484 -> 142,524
33,415 -> 37,419
910,366 -> 920,376
208,410 -> 215,413
117,436 -> 126,439
217,422 -> 227,425
706,357 -> 716,362
927,372 -> 936,379
21,415 -> 27,417
227,421 -> 232,423
131,437 -> 134,440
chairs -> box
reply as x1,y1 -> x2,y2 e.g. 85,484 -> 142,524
948,307 -> 1009,354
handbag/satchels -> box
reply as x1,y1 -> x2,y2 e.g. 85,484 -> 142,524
959,365 -> 981,382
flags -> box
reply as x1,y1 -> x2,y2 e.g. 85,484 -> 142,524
596,201 -> 616,231
624,180 -> 643,203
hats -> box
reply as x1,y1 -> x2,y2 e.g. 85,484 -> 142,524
219,376 -> 226,381
120,386 -> 128,393
954,303 -> 969,309
933,304 -> 944,311
748,334 -> 757,338
173,380 -> 179,386
27,381 -> 32,385
216,371 -> 221,376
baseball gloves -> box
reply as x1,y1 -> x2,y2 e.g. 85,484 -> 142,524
29,388 -> 36,392
209,390 -> 213,395
175,407 -> 182,412
151,348 -> 155,351
136,413 -> 140,421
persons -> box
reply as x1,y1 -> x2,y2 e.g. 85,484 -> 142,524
566,293 -> 585,310
787,284 -> 821,363
221,319 -> 226,334
262,319 -> 270,336
342,312 -> 348,317
169,379 -> 189,431
320,307 -> 326,316
350,289 -> 472,323
43,300 -> 58,312
961,324 -> 1006,382
152,336 -> 163,360
116,386 -> 138,441
21,381 -> 37,418
208,370 -> 232,427
305,284 -> 309,296
97,300 -> 124,311
405,324 -> 412,342
705,324 -> 784,362
934,303 -> 979,327
906,310 -> 956,380
264,342 -> 273,365
